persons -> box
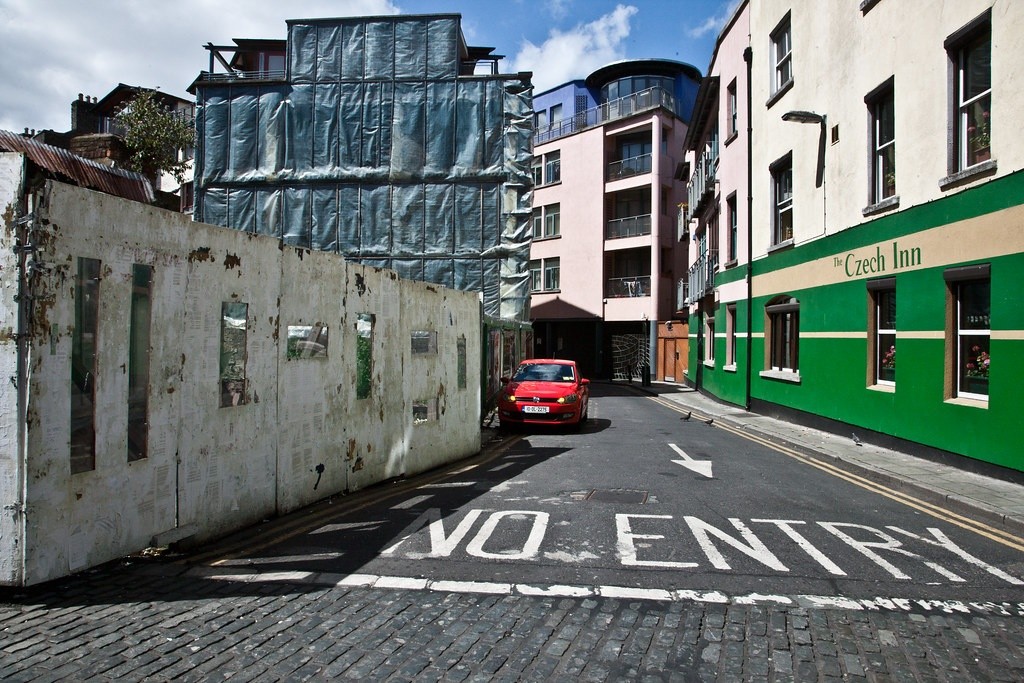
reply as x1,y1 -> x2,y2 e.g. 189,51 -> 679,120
551,367 -> 564,380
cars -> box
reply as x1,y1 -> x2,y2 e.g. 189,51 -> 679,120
496,359 -> 591,429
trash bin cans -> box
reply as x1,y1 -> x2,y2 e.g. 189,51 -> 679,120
641,365 -> 651,388
